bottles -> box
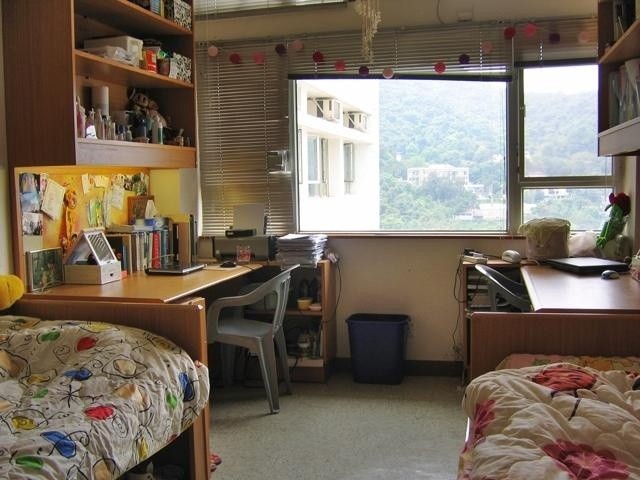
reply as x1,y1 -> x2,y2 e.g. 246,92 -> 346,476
75,103 -> 164,145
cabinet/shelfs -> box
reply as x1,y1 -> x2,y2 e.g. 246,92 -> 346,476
4,0 -> 200,169
246,259 -> 336,383
597,1 -> 639,157
459,258 -> 522,385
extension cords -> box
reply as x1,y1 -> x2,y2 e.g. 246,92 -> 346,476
459,251 -> 488,266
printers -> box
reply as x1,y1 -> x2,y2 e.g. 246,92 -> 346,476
197,203 -> 278,261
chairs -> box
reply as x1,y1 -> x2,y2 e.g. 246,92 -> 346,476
472,262 -> 535,312
206,262 -> 302,415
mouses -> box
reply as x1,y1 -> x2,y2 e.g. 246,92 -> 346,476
601,268 -> 620,280
219,260 -> 235,268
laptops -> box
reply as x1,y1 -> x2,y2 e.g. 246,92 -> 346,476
145,262 -> 207,275
545,255 -> 630,276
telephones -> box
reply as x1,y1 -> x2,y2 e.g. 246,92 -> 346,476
502,250 -> 521,263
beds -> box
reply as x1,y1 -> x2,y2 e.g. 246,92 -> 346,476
456,312 -> 639,480
0,300 -> 212,480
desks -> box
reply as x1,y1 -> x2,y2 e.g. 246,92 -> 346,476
23,259 -> 263,305
520,265 -> 639,311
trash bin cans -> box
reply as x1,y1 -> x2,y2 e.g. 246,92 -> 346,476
345,312 -> 410,385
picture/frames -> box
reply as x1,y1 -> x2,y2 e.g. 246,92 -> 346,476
128,195 -> 155,225
28,247 -> 64,293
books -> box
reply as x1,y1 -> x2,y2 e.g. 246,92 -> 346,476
467,269 -> 500,308
105,213 -> 208,275
601,0 -> 640,127
275,232 -> 327,271
